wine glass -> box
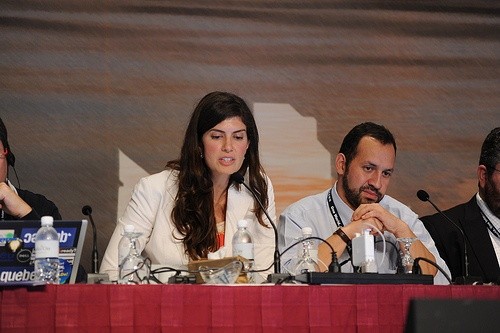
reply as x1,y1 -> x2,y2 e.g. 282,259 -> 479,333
396,238 -> 419,273
119,232 -> 149,284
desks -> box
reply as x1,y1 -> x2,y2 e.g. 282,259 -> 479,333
0,283 -> 500,333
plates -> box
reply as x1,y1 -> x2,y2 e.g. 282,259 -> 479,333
198,261 -> 242,284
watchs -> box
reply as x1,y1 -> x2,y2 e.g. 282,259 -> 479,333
333,225 -> 351,245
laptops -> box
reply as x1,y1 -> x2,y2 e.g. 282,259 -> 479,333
0,220 -> 88,285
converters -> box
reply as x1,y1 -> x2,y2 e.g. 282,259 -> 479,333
168,275 -> 198,284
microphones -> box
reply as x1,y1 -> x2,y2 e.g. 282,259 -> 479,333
415,188 -> 480,286
81,202 -> 119,281
231,170 -> 291,284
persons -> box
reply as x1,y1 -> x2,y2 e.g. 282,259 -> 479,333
277,121 -> 453,286
416,126 -> 500,286
98,92 -> 276,284
0,118 -> 88,284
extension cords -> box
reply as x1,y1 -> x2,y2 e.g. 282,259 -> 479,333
300,272 -> 437,285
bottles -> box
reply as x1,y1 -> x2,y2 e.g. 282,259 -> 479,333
34,216 -> 60,285
232,220 -> 254,284
297,227 -> 314,274
118,225 -> 140,284
